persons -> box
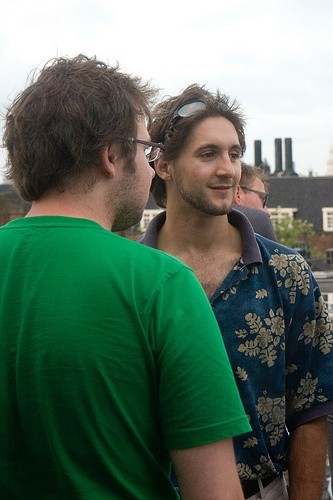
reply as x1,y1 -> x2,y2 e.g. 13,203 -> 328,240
0,51 -> 253,500
135,79 -> 333,500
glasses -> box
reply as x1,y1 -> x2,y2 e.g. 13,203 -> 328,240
107,134 -> 164,162
162,98 -> 206,135
240,184 -> 270,208
